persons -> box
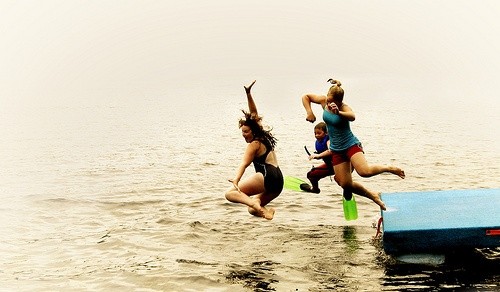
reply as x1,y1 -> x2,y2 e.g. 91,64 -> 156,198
225,79 -> 284,220
301,78 -> 406,211
299,121 -> 354,195
